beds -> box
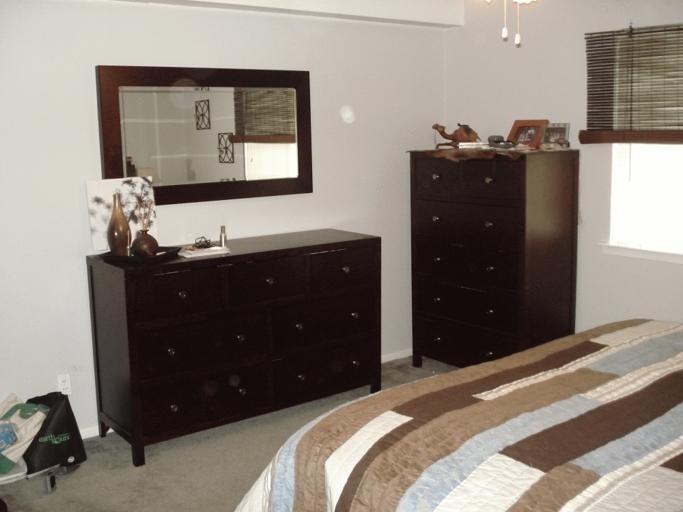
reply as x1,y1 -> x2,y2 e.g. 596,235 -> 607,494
234,320 -> 681,512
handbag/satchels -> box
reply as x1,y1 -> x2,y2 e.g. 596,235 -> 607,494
0,391 -> 87,476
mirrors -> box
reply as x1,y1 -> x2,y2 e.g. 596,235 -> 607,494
96,66 -> 313,205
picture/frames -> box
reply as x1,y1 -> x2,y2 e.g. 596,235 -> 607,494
544,123 -> 570,147
508,120 -> 547,150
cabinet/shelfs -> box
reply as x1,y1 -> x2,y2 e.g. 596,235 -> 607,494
410,150 -> 579,371
86,229 -> 381,472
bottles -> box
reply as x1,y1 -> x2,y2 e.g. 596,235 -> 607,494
218,225 -> 227,248
107,192 -> 132,257
124,156 -> 135,177
